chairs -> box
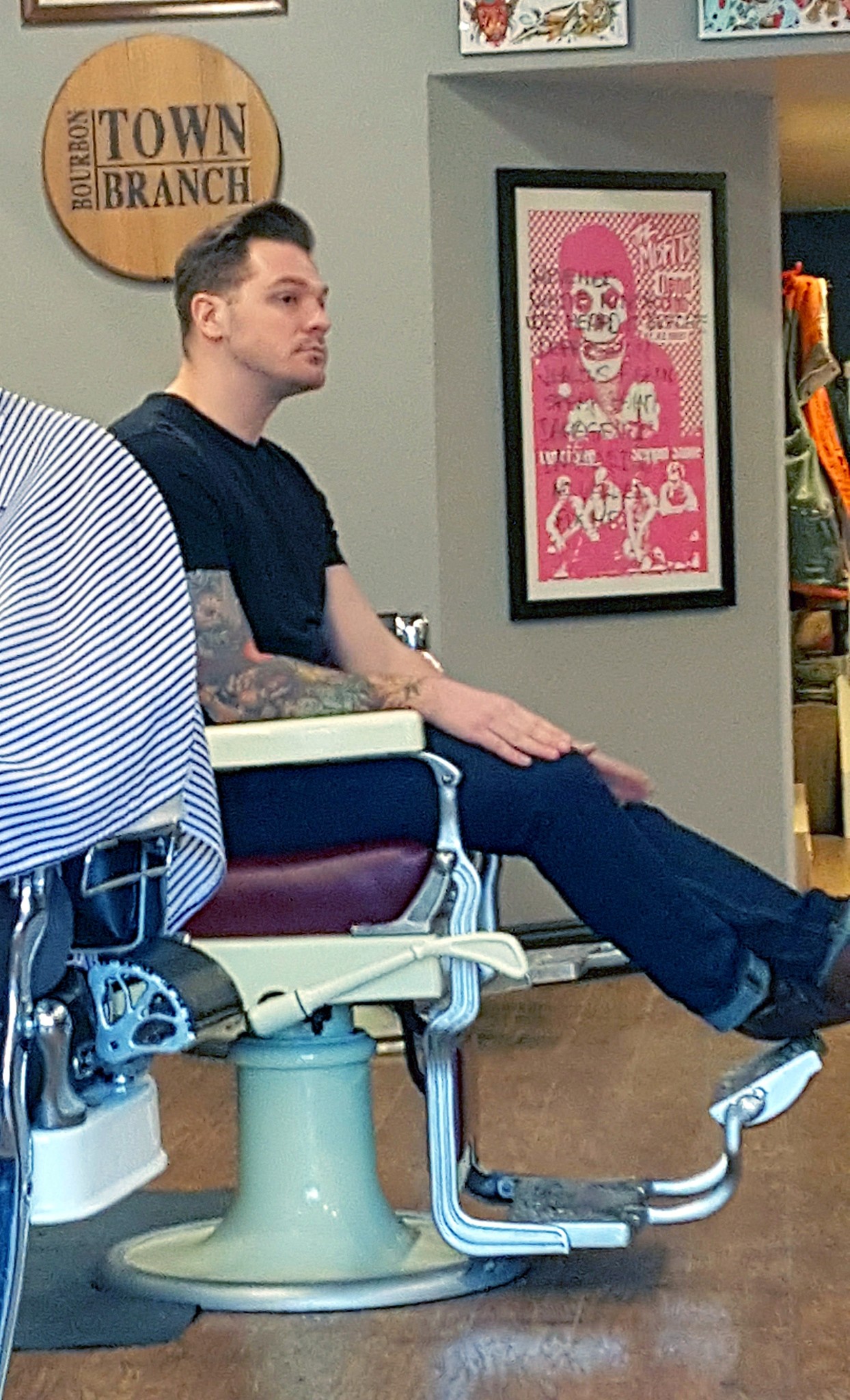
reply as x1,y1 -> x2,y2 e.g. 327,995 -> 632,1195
192,611 -> 824,1253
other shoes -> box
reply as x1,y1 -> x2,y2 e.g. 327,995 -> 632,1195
731,971 -> 850,1041
815,895 -> 850,1011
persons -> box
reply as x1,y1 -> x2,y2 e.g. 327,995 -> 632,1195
105,199 -> 850,1039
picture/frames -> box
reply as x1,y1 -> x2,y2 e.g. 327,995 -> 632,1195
495,165 -> 736,621
